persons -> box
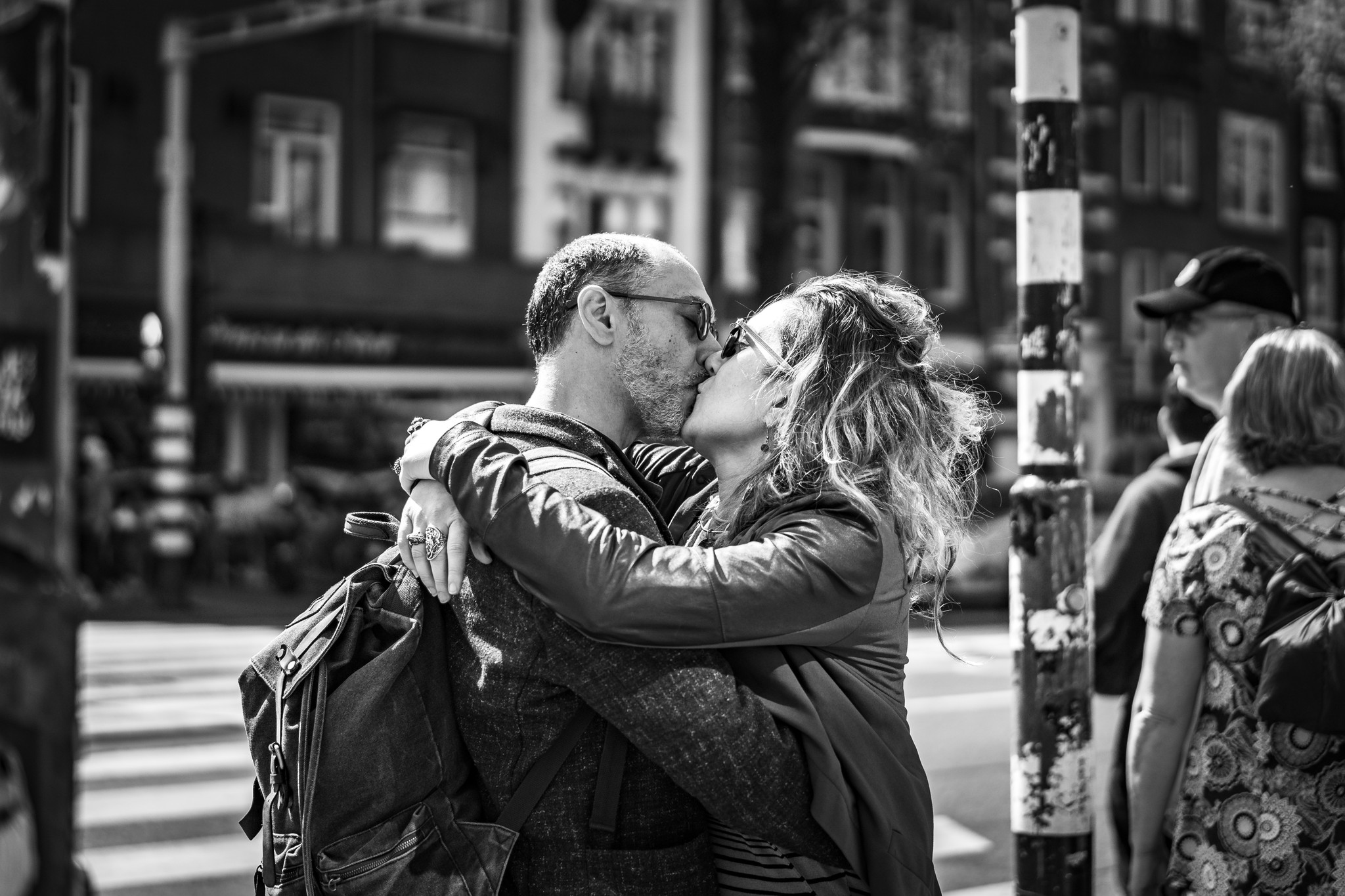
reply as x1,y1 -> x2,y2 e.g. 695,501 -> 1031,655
397,231 -> 942,896
1086,245 -> 1345,896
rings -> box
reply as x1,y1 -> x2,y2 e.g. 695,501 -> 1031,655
406,531 -> 425,549
425,524 -> 448,560
407,417 -> 432,435
392,456 -> 401,475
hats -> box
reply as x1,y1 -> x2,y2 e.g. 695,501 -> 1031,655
1132,249 -> 1302,325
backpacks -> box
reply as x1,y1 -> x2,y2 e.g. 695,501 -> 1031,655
238,450 -> 617,896
1204,496 -> 1345,739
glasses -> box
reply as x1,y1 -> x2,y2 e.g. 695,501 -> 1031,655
1163,311 -> 1261,333
566,291 -> 719,343
720,318 -> 798,377
